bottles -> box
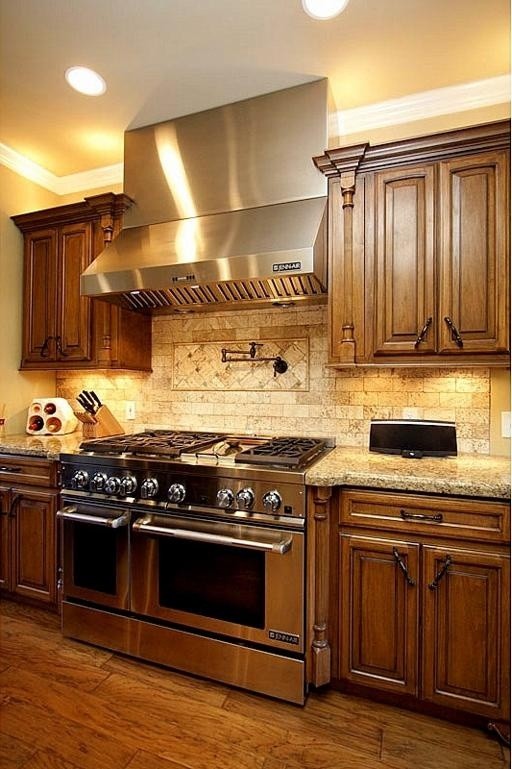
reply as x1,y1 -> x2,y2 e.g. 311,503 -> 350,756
27,404 -> 58,433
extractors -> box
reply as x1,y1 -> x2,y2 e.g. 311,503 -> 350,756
80,79 -> 332,315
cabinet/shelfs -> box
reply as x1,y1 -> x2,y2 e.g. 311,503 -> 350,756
312,117 -> 510,371
0,455 -> 61,614
10,191 -> 152,371
307,485 -> 510,734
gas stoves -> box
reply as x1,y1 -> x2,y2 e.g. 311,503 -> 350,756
60,427 -> 336,519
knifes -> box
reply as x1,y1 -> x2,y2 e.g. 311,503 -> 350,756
73,387 -> 101,414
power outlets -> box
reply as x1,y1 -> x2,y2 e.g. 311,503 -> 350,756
501,411 -> 510,438
125,401 -> 134,420
402,407 -> 420,419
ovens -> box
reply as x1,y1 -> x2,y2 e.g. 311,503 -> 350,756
54,491 -> 317,708
